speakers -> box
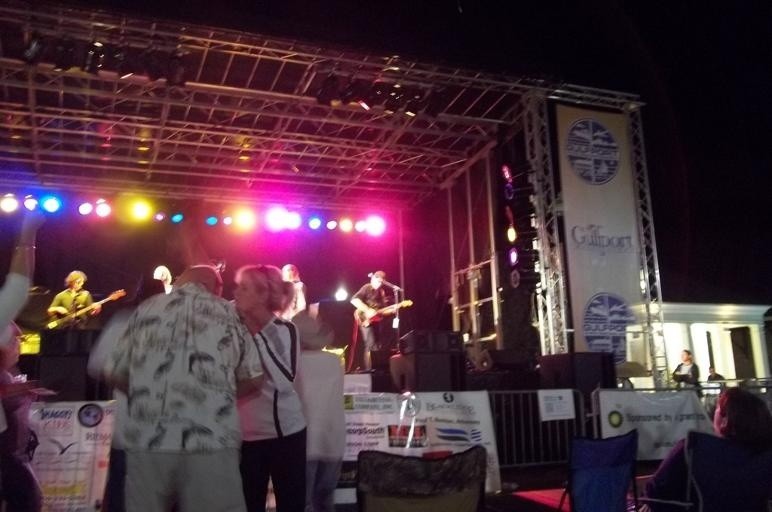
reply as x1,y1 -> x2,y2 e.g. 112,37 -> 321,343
18,355 -> 113,401
308,302 -> 354,350
40,329 -> 101,355
537,355 -> 575,389
398,330 -> 463,353
389,354 -> 452,392
573,352 -> 616,391
477,348 -> 539,389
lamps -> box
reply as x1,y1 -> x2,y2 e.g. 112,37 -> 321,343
315,67 -> 443,118
20,16 -> 186,87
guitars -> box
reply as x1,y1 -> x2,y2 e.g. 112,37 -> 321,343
354,300 -> 413,327
50,289 -> 127,330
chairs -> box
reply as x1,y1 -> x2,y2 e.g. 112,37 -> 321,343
627,431 -> 772,512
356,445 -> 487,512
511,428 -> 638,512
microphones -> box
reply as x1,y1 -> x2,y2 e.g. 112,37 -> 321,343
368,272 -> 384,285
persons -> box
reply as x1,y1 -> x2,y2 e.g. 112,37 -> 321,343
347,269 -> 399,370
642,386 -> 771,511
280,264 -> 308,321
47,271 -> 101,329
0,204 -> 47,511
88,265 -> 264,511
670,348 -> 704,401
705,365 -> 727,397
292,310 -> 347,511
229,265 -> 308,511
